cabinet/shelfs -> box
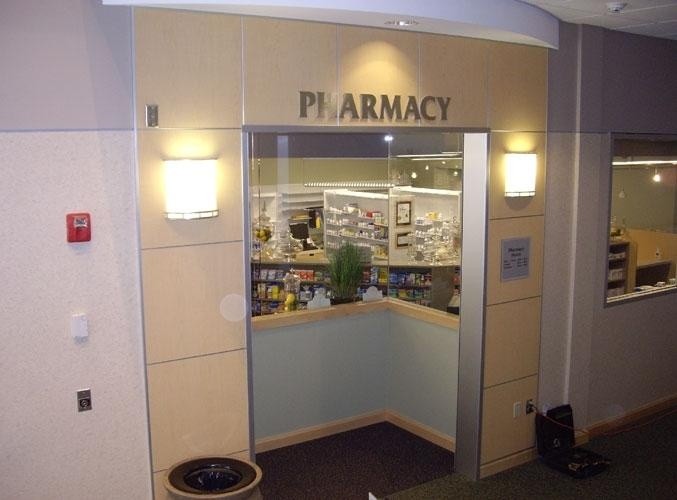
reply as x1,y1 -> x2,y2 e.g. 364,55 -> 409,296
606,230 -> 637,294
251,182 -> 460,314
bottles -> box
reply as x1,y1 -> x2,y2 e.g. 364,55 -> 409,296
253,201 -> 461,316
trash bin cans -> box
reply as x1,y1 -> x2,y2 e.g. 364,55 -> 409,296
163,454 -> 263,499
